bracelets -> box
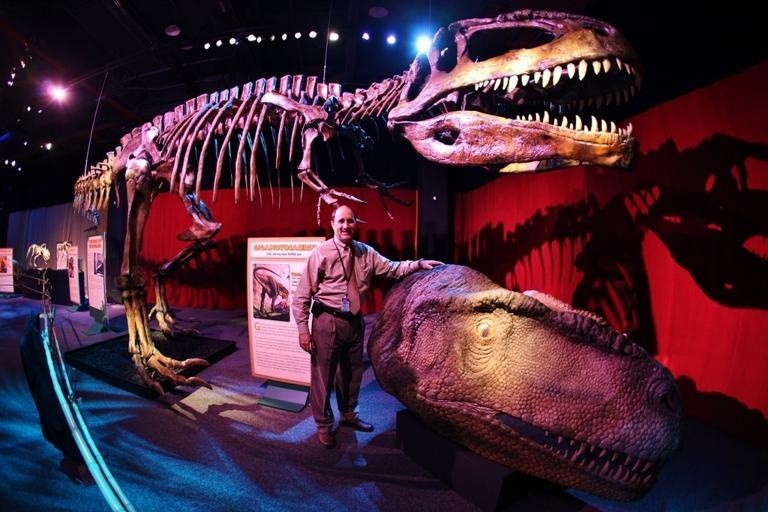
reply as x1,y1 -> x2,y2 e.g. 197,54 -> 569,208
418,258 -> 424,267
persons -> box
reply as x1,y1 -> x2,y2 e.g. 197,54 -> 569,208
291,206 -> 447,448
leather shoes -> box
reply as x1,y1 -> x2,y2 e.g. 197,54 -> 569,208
346,419 -> 374,431
318,431 -> 334,449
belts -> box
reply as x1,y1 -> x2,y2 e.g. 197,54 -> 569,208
322,304 -> 361,321
